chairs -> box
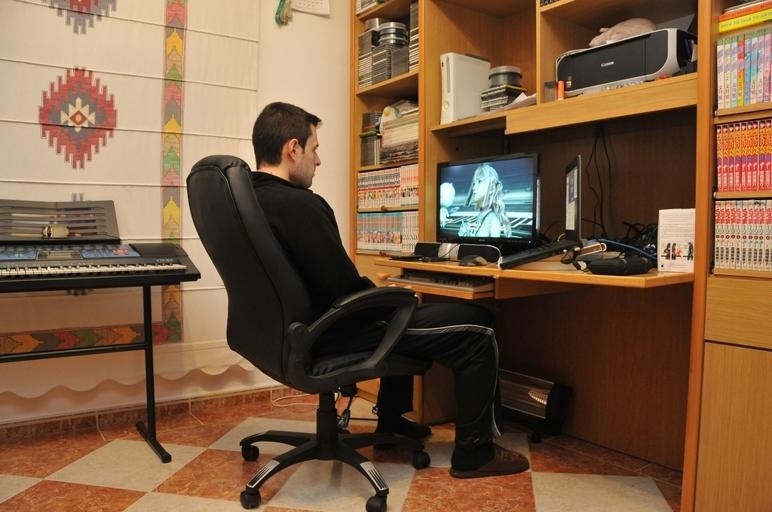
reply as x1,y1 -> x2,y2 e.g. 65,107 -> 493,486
185,154 -> 432,512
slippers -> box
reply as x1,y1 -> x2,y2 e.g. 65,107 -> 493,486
374,419 -> 432,449
449,443 -> 530,478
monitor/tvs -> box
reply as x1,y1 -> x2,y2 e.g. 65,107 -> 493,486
436,150 -> 541,253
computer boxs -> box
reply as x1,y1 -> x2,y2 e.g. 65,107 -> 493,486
555,26 -> 698,99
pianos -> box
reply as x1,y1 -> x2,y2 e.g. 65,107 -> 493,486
2,238 -> 200,292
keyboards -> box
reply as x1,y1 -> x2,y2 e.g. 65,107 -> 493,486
387,272 -> 494,294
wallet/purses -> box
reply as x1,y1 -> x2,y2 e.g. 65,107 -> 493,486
589,257 -> 648,276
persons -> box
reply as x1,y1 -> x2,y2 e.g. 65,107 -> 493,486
457,165 -> 512,238
248,102 -> 529,478
663,242 -> 693,260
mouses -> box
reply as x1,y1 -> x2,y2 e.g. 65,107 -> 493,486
460,255 -> 487,266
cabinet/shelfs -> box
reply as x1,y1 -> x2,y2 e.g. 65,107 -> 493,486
350,1 -> 423,255
702,5 -> 770,277
350,258 -> 417,411
695,280 -> 771,512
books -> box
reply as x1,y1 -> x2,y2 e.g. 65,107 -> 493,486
357,163 -> 419,209
714,200 -> 772,272
716,117 -> 772,192
357,1 -> 419,90
480,85 -> 528,112
715,0 -> 771,110
360,100 -> 419,166
356,211 -> 419,253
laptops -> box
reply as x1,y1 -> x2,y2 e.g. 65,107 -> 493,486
498,154 -> 582,269
